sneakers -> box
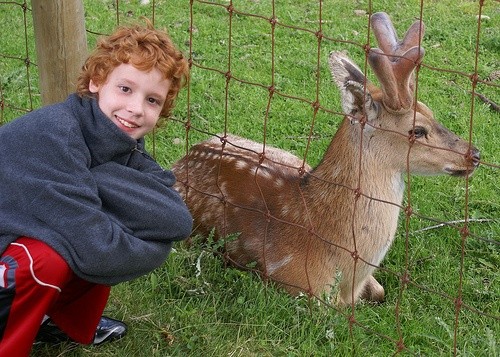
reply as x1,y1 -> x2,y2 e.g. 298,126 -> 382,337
37,313 -> 127,346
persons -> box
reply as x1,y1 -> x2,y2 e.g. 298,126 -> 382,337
1,15 -> 193,357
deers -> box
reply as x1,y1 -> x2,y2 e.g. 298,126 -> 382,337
167,12 -> 481,305
